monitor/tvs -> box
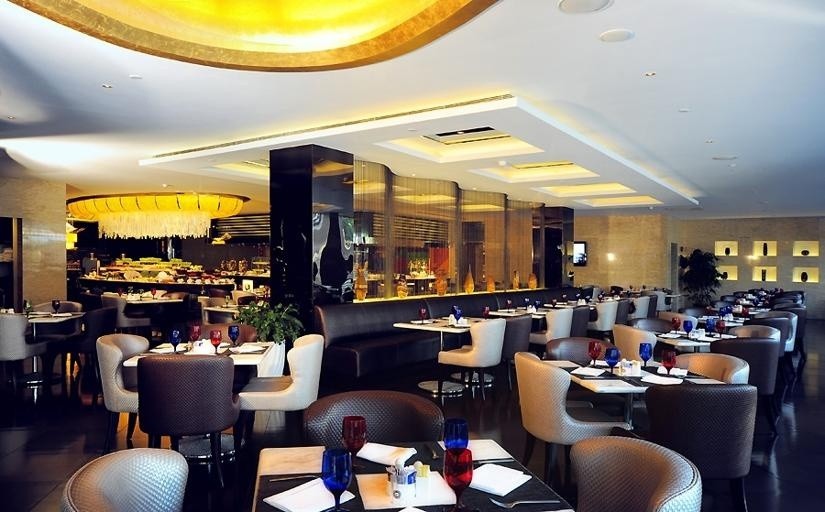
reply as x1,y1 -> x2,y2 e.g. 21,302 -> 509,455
574,241 -> 586,267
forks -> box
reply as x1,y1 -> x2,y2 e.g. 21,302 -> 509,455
490,495 -> 561,509
424,441 -> 442,460
269,473 -> 322,482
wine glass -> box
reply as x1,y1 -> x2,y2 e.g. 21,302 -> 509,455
417,285 -> 646,328
442,447 -> 472,512
589,340 -> 602,369
605,346 -> 620,376
169,329 -> 180,353
118,286 -> 156,299
639,342 -> 654,371
341,416 -> 368,469
228,325 -> 239,347
210,330 -> 222,355
51,299 -> 61,314
23,299 -> 31,319
663,350 -> 676,377
671,298 -> 760,340
321,450 -> 354,512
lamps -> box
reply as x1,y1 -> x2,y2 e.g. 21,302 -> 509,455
64,191 -> 256,241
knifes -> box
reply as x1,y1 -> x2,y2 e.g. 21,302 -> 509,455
472,457 -> 514,466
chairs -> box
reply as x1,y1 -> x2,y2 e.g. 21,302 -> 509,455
0,256 -> 818,512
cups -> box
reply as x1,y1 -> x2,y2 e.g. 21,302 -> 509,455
176,278 -> 234,284
223,296 -> 230,309
388,468 -> 419,502
187,325 -> 200,342
442,416 -> 469,457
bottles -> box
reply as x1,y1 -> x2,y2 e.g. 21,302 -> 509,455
354,266 -> 539,301
